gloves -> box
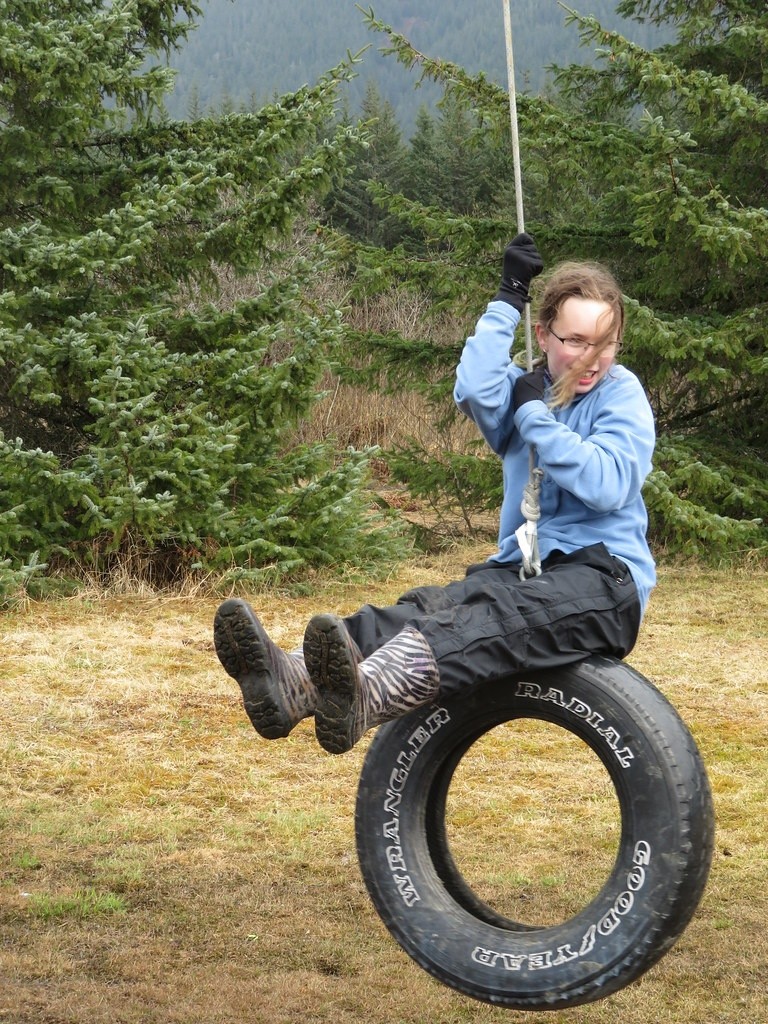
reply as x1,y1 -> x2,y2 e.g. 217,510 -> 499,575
494,233 -> 543,312
513,366 -> 545,415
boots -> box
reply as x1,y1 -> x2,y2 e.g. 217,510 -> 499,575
302,613 -> 441,754
214,598 -> 364,740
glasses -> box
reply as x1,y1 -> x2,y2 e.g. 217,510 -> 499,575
547,327 -> 624,357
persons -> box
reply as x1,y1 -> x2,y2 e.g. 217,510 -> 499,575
213,234 -> 656,753
